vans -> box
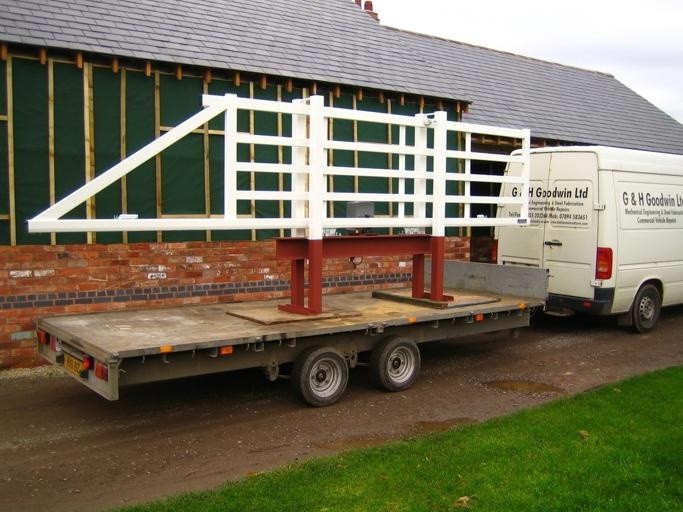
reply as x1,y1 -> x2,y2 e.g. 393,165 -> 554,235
493,145 -> 683,331
